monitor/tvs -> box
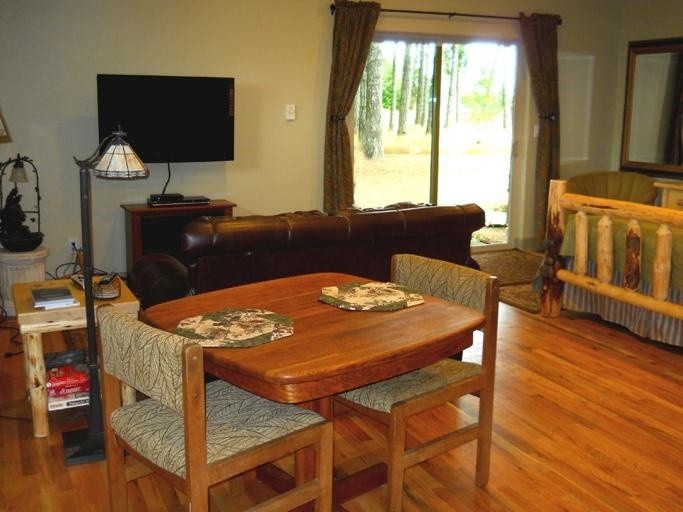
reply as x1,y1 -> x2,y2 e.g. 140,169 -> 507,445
96,73 -> 235,164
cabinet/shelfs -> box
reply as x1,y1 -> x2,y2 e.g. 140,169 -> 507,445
653,181 -> 683,211
121,199 -> 237,287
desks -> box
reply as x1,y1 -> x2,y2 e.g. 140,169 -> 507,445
11,275 -> 140,439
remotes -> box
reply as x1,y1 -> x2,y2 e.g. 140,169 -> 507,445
99,271 -> 115,284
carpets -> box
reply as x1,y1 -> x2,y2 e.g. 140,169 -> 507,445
471,248 -> 545,314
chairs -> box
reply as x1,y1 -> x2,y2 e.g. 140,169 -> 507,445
95,302 -> 332,512
328,253 -> 498,511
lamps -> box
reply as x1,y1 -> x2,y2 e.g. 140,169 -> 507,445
0,152 -> 43,252
60,122 -> 173,467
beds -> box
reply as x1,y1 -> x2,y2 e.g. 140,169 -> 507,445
533,170 -> 683,349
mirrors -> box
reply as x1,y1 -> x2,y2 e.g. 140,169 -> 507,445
617,36 -> 681,177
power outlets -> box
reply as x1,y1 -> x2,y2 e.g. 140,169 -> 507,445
68,238 -> 80,256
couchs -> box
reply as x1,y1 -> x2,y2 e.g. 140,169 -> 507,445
131,201 -> 484,308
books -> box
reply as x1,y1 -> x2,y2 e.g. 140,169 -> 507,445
44,348 -> 102,412
30,285 -> 81,311
